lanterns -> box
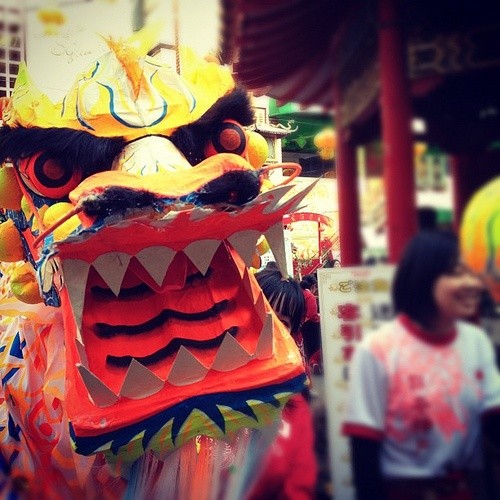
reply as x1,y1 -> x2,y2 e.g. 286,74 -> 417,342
314,125 -> 336,161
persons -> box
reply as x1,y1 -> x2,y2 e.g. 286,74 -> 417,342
254,255 -> 344,373
336,224 -> 500,500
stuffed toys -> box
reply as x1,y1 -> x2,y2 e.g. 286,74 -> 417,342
0,0 -> 321,500
